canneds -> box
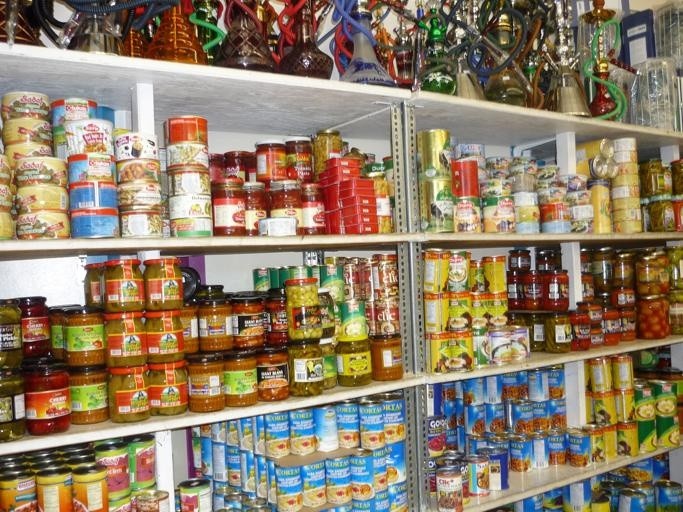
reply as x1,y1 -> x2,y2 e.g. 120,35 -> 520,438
3,92 -> 392,238
417,115 -> 683,233
428,356 -> 682,511
1,255 -> 406,441
1,392 -> 409,512
425,241 -> 683,372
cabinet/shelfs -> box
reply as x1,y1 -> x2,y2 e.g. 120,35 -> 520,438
0,43 -> 681,509
